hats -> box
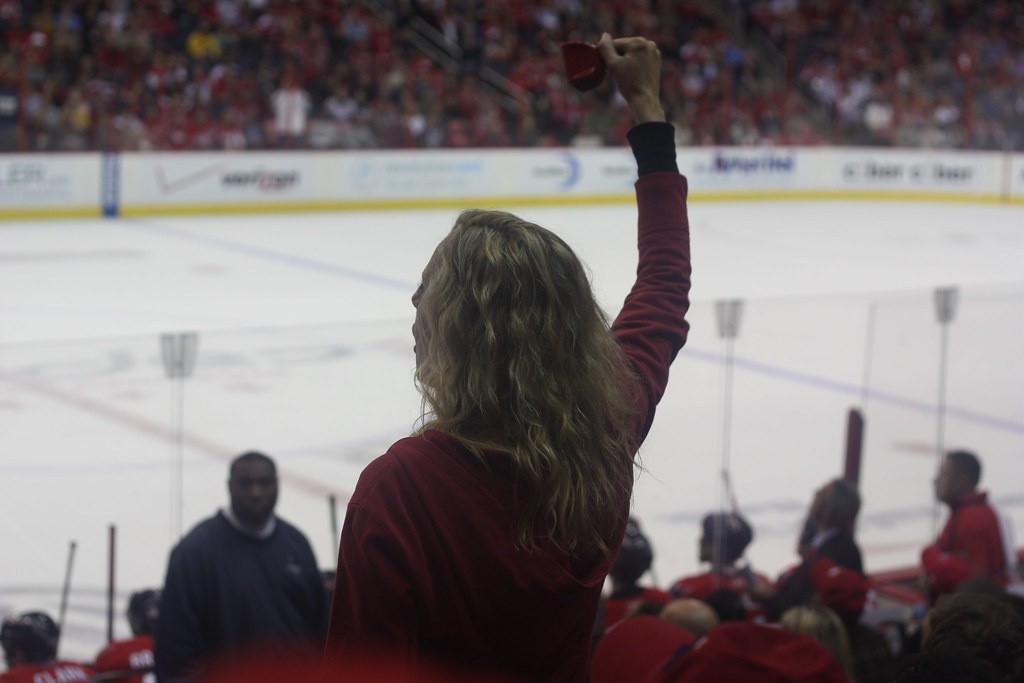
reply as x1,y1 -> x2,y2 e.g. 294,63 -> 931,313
811,558 -> 872,609
922,548 -> 967,595
589,611 -> 849,683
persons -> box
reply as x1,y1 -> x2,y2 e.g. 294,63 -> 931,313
334,33 -> 691,682
3,5 -> 1024,680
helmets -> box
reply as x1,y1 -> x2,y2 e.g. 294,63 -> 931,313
1,613 -> 60,661
128,591 -> 161,633
